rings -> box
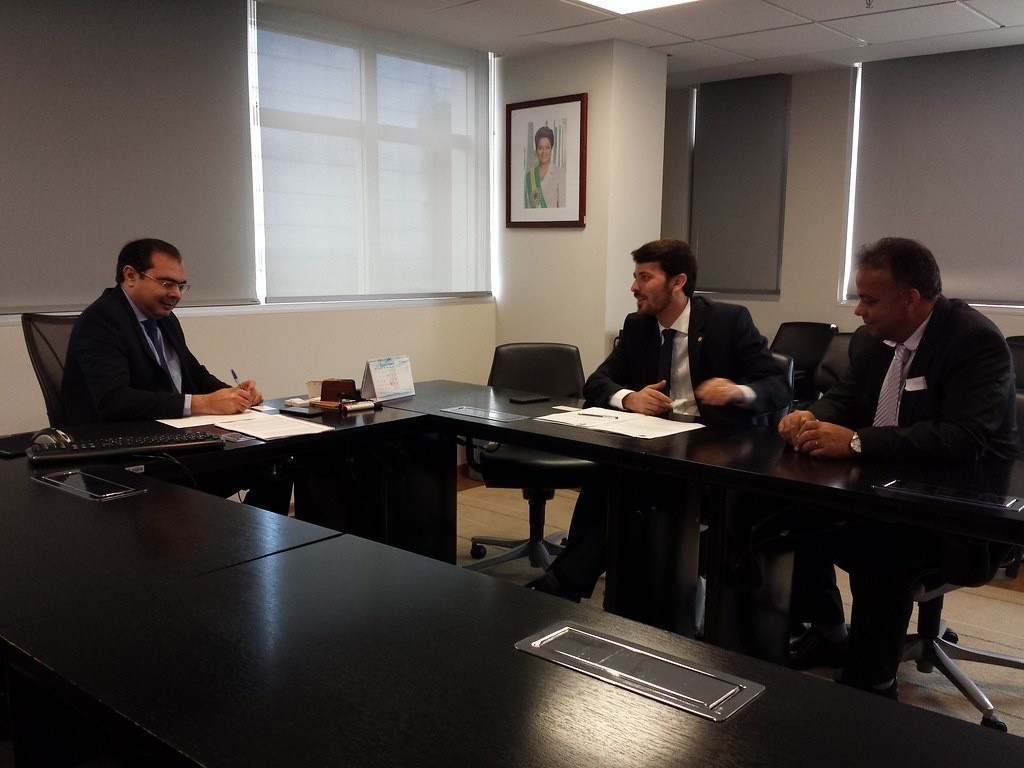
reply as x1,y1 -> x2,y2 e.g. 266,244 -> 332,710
815,439 -> 820,448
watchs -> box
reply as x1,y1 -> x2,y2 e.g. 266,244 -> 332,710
848,432 -> 861,455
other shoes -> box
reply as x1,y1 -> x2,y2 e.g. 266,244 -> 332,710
788,627 -> 848,665
525,574 -> 565,595
842,668 -> 900,700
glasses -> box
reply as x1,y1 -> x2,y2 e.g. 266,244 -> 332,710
137,270 -> 192,296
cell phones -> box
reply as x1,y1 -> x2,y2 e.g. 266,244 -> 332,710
509,394 -> 551,403
279,406 -> 326,417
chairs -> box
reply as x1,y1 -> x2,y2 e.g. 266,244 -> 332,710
700,309 -> 1024,729
20,312 -> 77,431
459,343 -> 584,574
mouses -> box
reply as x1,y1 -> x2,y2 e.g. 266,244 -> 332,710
31,427 -> 73,445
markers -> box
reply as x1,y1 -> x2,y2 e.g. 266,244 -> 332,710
339,401 -> 383,414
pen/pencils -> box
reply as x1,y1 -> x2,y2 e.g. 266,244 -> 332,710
231,369 -> 240,385
578,413 -> 619,420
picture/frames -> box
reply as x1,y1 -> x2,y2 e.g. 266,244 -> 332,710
508,92 -> 588,228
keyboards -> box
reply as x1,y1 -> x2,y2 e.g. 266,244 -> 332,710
25,431 -> 225,464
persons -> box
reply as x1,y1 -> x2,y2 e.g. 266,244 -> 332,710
769,237 -> 1019,701
525,238 -> 794,603
524,126 -> 566,209
56,238 -> 292,517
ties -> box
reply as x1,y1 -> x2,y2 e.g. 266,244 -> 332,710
142,319 -> 182,396
871,340 -> 909,432
656,328 -> 678,408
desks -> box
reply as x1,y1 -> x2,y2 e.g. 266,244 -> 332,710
2,378 -> 1024,768
